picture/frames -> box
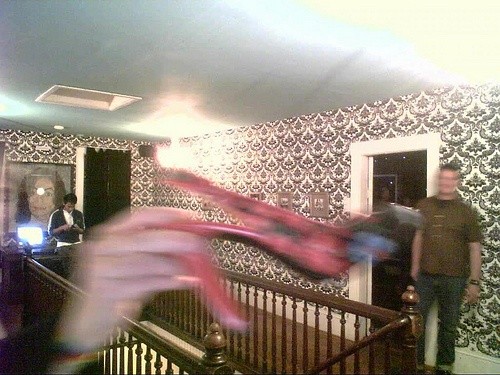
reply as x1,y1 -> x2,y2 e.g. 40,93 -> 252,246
2,160 -> 76,238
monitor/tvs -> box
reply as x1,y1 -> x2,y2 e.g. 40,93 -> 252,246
16,225 -> 43,245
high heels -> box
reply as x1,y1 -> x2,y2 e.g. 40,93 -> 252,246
160,169 -> 426,330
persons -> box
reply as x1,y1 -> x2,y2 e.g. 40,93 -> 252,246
410,164 -> 482,374
48,195 -> 86,257
0,206 -> 208,375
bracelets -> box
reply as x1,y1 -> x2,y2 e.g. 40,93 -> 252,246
468,278 -> 479,285
